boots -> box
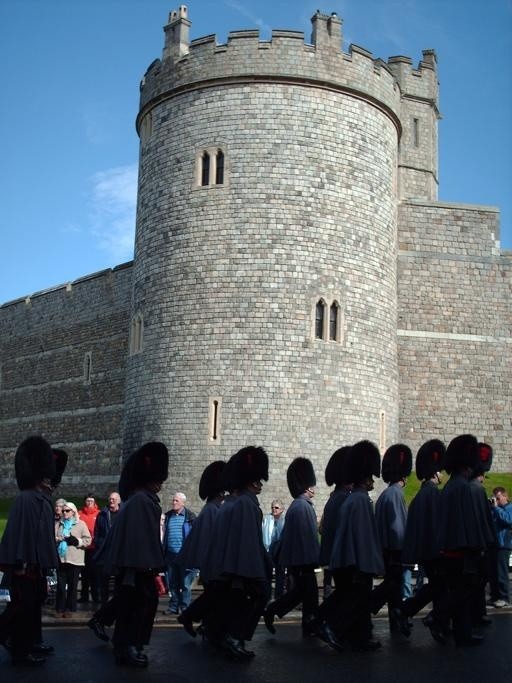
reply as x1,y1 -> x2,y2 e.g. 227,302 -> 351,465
324,585 -> 331,598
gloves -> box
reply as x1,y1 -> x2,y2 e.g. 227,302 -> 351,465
66,535 -> 78,546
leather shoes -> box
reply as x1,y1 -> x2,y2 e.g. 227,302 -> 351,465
302,618 -> 381,652
86,618 -> 109,642
23,653 -> 46,666
392,607 -> 411,637
262,608 -> 276,634
422,614 -> 492,646
177,614 -> 197,638
199,625 -> 255,658
33,644 -> 54,653
390,622 -> 413,633
119,645 -> 147,665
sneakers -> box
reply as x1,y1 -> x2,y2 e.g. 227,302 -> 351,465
163,609 -> 176,615
55,597 -> 89,618
487,598 -> 509,607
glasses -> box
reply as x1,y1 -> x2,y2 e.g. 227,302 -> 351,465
62,510 -> 71,513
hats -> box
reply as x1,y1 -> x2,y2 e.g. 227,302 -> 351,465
15,437 -> 67,490
325,446 -> 351,486
216,446 -> 268,491
382,444 -> 412,483
199,461 -> 226,499
287,458 -> 316,498
416,439 -> 446,480
478,443 -> 492,471
344,440 -> 380,485
119,442 -> 168,502
445,435 -> 478,475
